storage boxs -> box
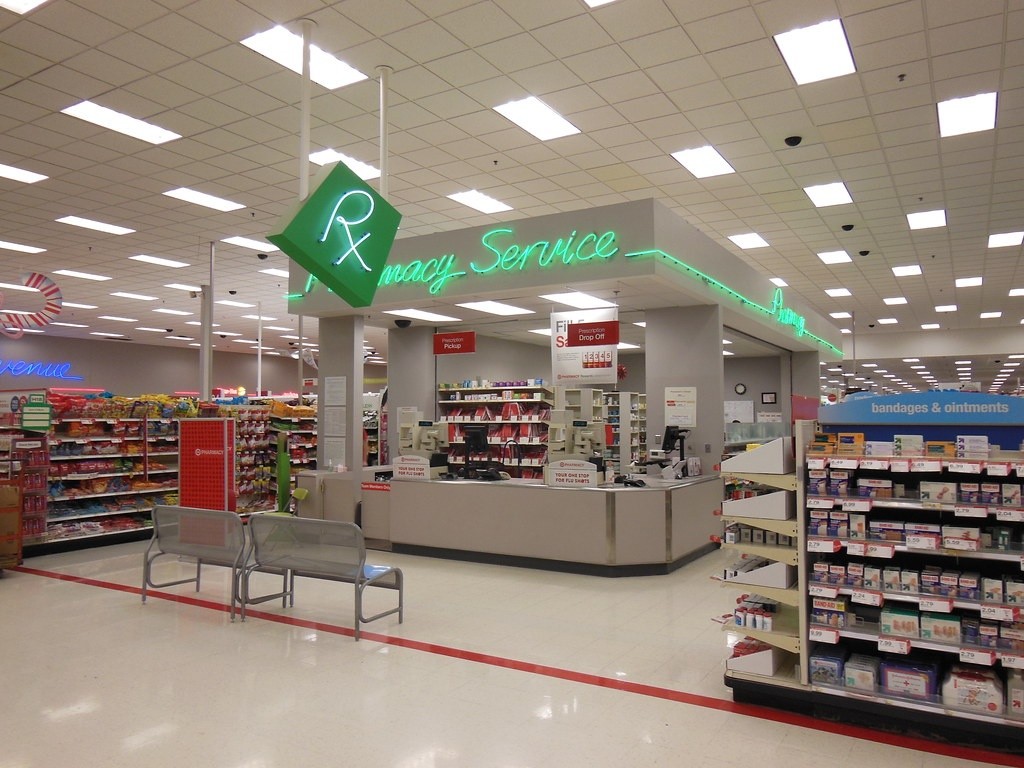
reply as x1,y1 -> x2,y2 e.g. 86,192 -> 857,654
0,485 -> 22,571
807,432 -> 1024,719
721,436 -> 795,678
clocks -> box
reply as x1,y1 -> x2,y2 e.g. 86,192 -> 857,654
734,383 -> 746,395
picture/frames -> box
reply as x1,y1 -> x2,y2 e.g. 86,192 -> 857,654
761,392 -> 777,405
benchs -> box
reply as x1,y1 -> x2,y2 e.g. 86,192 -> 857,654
141,504 -> 289,624
241,513 -> 404,642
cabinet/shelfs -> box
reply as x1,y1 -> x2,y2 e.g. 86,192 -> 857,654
717,418 -> 817,716
806,449 -> 1024,757
564,388 -> 647,474
268,409 -> 380,511
438,386 -> 557,467
0,417 -> 179,559
